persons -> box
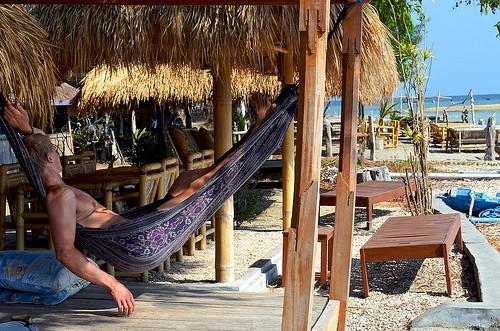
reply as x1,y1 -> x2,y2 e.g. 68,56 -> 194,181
443,109 -> 469,122
3,91 -> 277,317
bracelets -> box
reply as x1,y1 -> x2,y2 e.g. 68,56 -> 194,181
31,126 -> 34,134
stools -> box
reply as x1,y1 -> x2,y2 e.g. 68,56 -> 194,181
282,228 -> 334,290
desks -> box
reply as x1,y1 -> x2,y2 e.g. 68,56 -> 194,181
360,213 -> 463,298
319,180 -> 416,230
15,166 -> 186,277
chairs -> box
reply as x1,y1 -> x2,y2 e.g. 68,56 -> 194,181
0,149 -> 215,282
